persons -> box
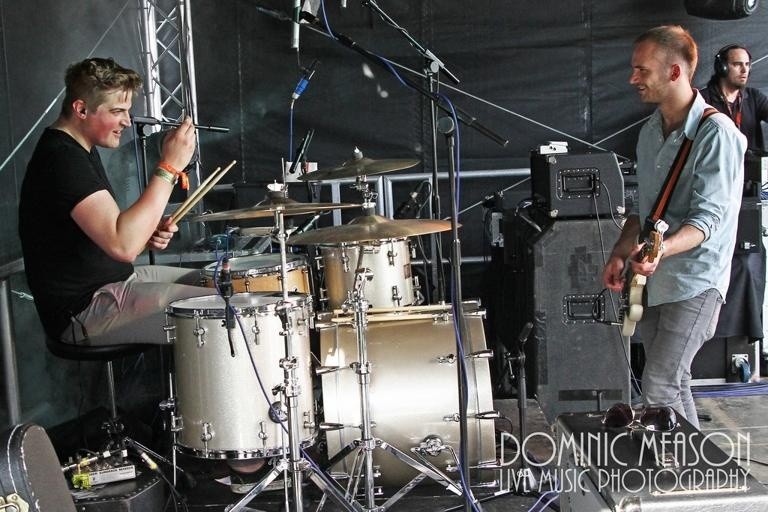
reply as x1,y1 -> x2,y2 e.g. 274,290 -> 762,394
18,59 -> 310,496
601,26 -> 748,433
699,43 -> 768,198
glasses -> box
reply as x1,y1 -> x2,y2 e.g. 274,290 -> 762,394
600,402 -> 681,433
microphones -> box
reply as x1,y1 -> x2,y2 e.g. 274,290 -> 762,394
288,129 -> 313,175
392,187 -> 419,219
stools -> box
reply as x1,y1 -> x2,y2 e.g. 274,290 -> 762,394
43,337 -> 194,505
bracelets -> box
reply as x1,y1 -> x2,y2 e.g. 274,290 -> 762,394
152,166 -> 180,187
158,161 -> 189,190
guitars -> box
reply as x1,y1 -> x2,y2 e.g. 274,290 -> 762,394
619,220 -> 667,336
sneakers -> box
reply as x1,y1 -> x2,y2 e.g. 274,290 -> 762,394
229,464 -> 293,493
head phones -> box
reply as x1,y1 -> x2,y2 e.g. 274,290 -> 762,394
713,44 -> 735,77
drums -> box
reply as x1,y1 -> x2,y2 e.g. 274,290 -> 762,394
206,253 -> 311,295
322,241 -> 418,309
168,291 -> 319,461
315,300 -> 496,485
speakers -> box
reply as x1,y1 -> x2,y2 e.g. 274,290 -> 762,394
515,197 -> 633,424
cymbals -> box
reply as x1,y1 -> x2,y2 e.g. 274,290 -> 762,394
185,201 -> 360,224
287,219 -> 461,246
298,159 -> 422,182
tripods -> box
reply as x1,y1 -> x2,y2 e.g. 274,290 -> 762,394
317,298 -> 483,512
442,363 -> 559,511
227,344 -> 356,512
